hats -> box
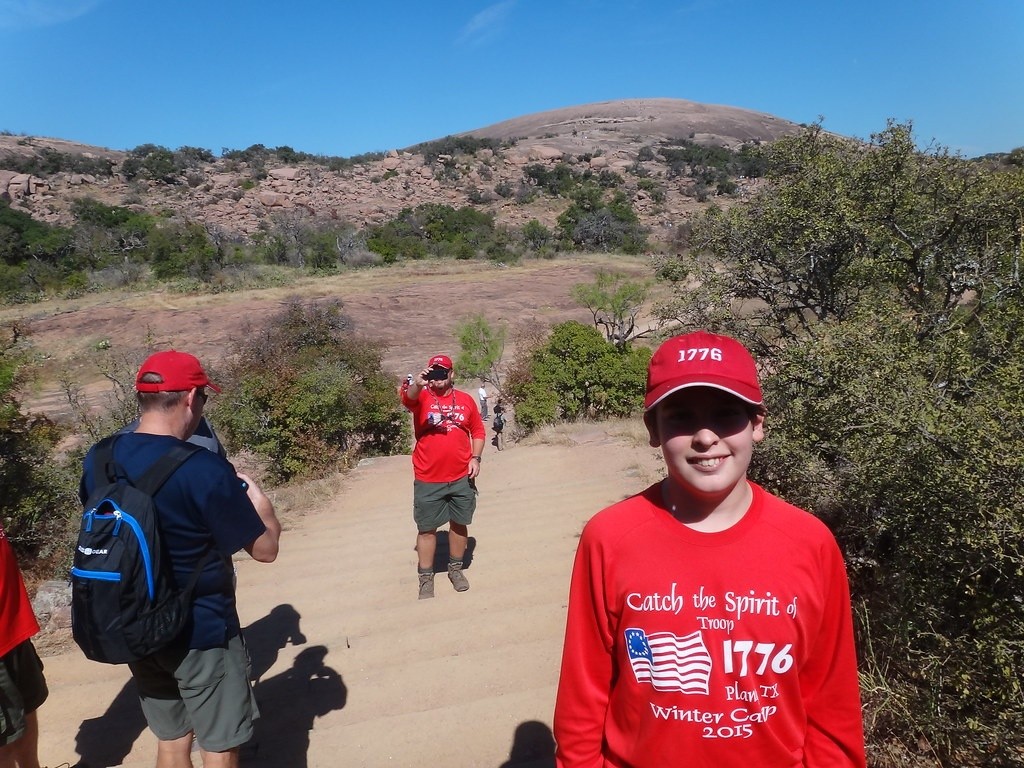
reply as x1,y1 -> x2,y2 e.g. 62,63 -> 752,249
408,374 -> 412,378
429,355 -> 452,369
645,331 -> 763,411
136,350 -> 222,393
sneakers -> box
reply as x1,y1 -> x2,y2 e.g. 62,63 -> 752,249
448,564 -> 469,592
419,574 -> 434,600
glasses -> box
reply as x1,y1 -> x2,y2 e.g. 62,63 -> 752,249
197,392 -> 208,404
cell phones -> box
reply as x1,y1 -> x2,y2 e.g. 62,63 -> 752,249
426,369 -> 448,380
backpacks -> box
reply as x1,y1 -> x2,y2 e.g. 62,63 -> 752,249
494,413 -> 503,431
71,436 -> 209,665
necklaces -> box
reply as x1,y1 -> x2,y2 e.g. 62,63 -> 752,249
427,386 -> 455,414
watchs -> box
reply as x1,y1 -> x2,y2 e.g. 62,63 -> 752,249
472,456 -> 481,463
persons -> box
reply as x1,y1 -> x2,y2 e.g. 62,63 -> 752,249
400,355 -> 506,600
551,330 -> 866,768
0,523 -> 50,768
81,348 -> 282,768
478,383 -> 494,420
493,399 -> 508,451
